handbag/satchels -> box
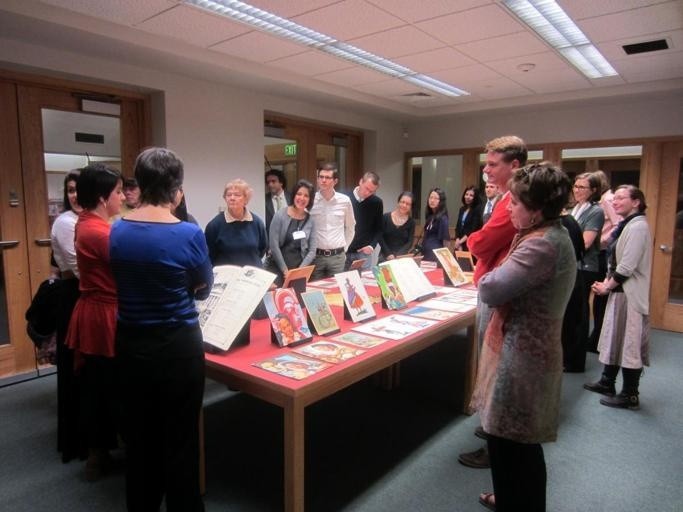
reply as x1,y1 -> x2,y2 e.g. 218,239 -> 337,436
34,333 -> 57,365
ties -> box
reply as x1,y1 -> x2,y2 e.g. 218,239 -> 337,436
487,201 -> 492,222
276,197 -> 281,210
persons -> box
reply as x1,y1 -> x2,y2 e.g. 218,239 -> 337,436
106,147 -> 216,512
49,165 -> 144,477
202,165 -> 384,290
566,170 -> 652,411
422,188 -> 451,261
457,134 -> 529,469
477,162 -> 578,511
482,182 -> 500,226
380,192 -> 415,262
453,184 -> 482,260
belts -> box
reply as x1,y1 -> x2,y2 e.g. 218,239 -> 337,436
316,247 -> 344,257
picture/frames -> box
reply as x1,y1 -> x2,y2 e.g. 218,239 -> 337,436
257,245 -> 474,349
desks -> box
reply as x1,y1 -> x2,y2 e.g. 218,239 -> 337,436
192,267 -> 478,511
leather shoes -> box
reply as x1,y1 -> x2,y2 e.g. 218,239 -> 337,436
458,444 -> 491,469
584,381 -> 616,397
599,390 -> 640,409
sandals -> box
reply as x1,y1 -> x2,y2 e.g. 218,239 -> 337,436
479,492 -> 495,507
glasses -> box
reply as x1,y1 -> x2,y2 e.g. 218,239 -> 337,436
571,184 -> 590,193
